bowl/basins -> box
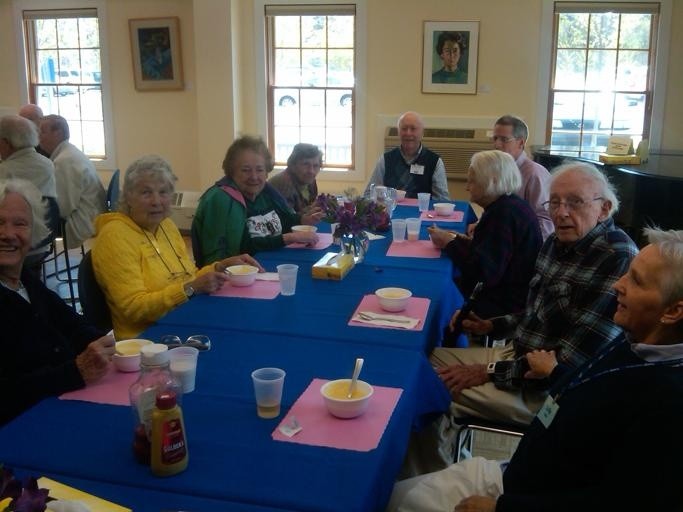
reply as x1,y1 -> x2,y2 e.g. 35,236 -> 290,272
375,287 -> 415,314
433,203 -> 456,216
396,190 -> 406,202
320,378 -> 374,421
223,265 -> 259,287
109,338 -> 154,374
291,225 -> 317,234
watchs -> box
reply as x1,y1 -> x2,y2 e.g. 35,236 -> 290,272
487,362 -> 496,382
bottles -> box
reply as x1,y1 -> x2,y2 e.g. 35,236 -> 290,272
363,184 -> 396,217
125,342 -> 185,466
149,390 -> 190,477
336,195 -> 345,206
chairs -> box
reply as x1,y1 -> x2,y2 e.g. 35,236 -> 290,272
56,169 -> 122,285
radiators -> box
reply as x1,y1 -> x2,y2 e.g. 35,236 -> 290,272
383,127 -> 497,184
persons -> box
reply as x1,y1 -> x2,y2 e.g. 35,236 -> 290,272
386,227 -> 683,512
432,31 -> 468,84
427,149 -> 543,320
363,111 -> 450,201
403,161 -> 639,478
468,115 -> 556,242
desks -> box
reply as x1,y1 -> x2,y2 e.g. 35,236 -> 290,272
0,197 -> 473,510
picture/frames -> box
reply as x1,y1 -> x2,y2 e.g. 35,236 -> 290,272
126,13 -> 187,93
417,17 -> 482,98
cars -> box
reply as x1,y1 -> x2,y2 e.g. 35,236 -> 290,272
80,71 -> 101,94
275,65 -> 352,107
42,67 -> 80,96
550,64 -> 632,150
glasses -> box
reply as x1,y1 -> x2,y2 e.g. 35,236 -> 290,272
540,197 -> 604,213
160,335 -> 211,353
488,136 -> 515,143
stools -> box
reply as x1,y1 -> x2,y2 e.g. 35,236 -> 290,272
448,415 -> 523,470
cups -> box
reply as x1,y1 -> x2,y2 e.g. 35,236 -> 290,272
406,217 -> 420,242
390,218 -> 406,244
250,366 -> 286,420
166,347 -> 200,393
417,192 -> 431,213
276,263 -> 298,297
